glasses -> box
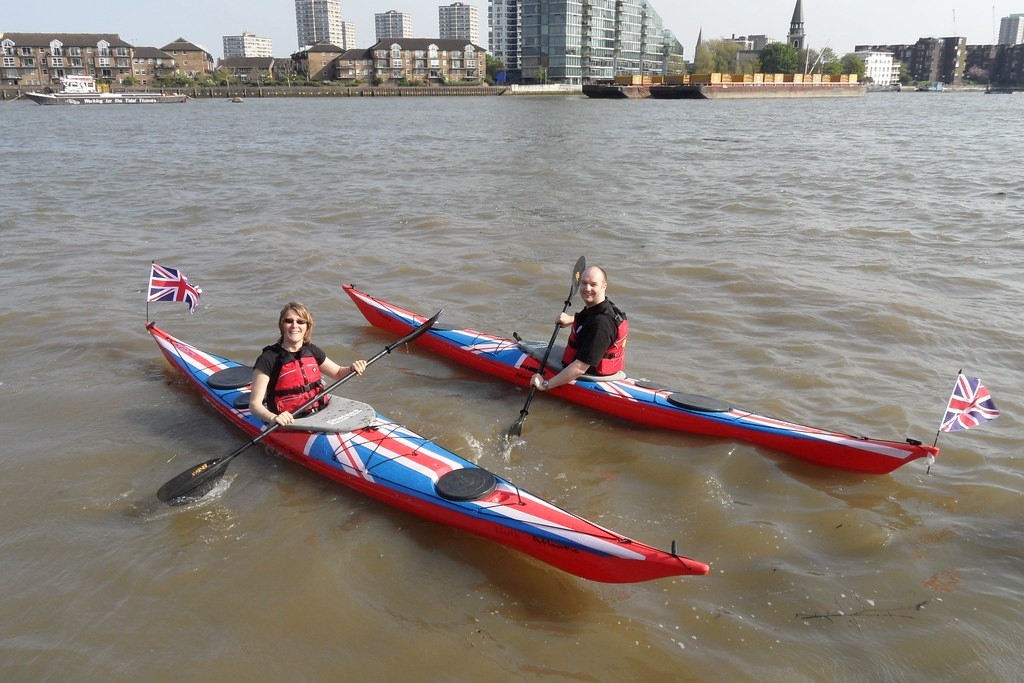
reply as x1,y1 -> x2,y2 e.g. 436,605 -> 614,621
285,319 -> 308,324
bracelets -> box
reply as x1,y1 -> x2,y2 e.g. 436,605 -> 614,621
350,366 -> 352,371
543,380 -> 549,389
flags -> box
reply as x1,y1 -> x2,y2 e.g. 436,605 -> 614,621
150,265 -> 201,313
940,374 -> 1002,433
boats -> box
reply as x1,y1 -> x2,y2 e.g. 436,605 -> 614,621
919,81 -> 944,91
650,84 -> 867,99
144,321 -> 710,585
985,88 -> 1012,94
23,75 -> 187,105
341,282 -> 938,475
582,83 -> 652,98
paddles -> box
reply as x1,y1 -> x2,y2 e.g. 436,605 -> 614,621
157,310 -> 446,505
502,255 -> 586,446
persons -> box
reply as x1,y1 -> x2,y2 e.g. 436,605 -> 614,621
530,266 -> 627,391
248,302 -> 366,428
